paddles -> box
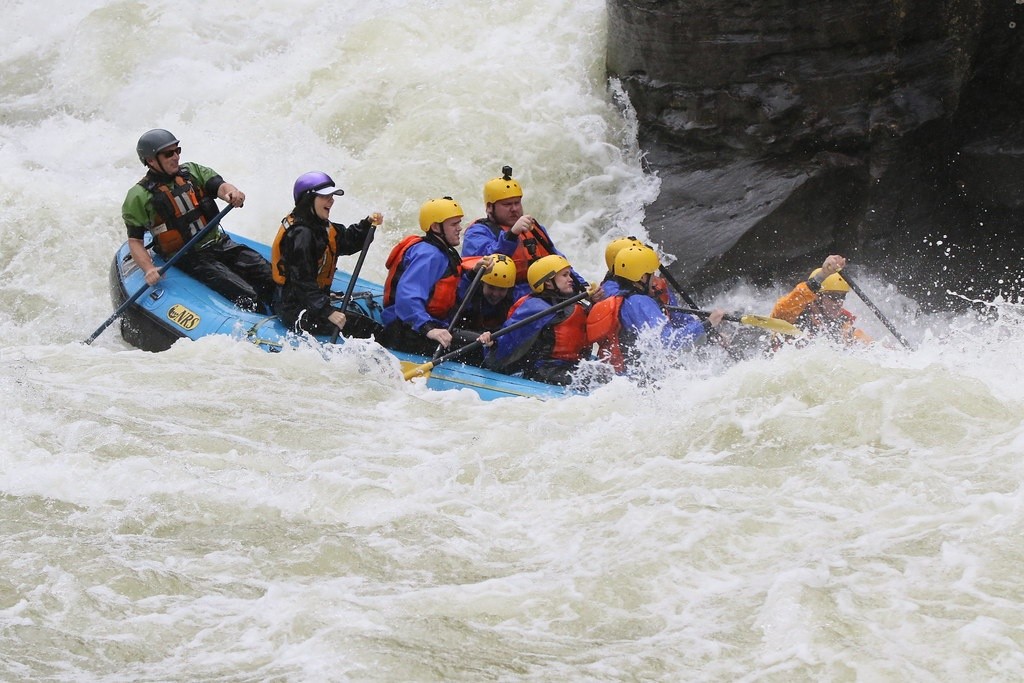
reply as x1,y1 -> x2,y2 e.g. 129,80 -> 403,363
392,265 -> 490,386
659,264 -> 725,341
656,301 -> 802,338
831,262 -> 912,355
394,281 -> 598,382
85,197 -> 241,343
527,221 -> 594,302
323,215 -> 380,363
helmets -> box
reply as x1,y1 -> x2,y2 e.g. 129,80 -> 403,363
526,254 -> 569,293
480,253 -> 516,288
809,267 -> 850,292
614,245 -> 661,282
419,198 -> 463,232
605,237 -> 645,275
483,177 -> 523,208
294,171 -> 344,206
137,128 -> 180,167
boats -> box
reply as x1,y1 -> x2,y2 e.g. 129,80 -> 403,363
108,228 -> 677,404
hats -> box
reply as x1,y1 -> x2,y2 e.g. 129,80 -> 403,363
311,185 -> 343,197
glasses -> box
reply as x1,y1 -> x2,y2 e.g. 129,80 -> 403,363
821,292 -> 847,301
157,147 -> 181,159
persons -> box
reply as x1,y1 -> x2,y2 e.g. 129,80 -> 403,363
763,256 -> 873,358
456,253 -> 523,348
480,256 -> 605,385
124,131 -> 281,313
380,196 -> 482,365
271,171 -> 389,346
461,167 -> 593,301
587,246 -> 725,383
590,237 -> 694,327
